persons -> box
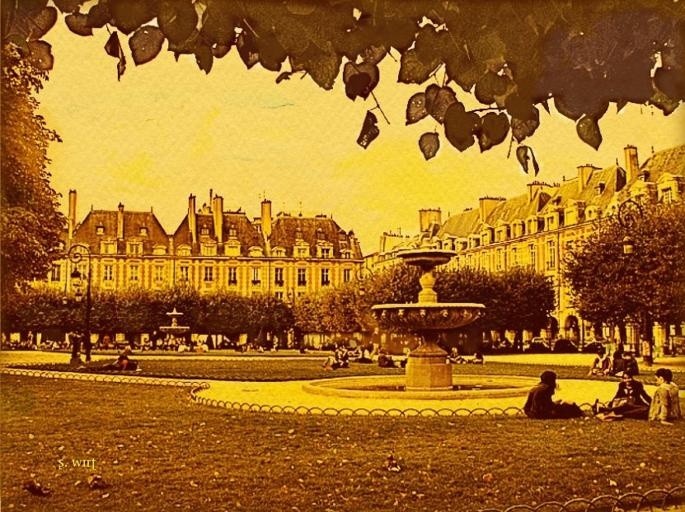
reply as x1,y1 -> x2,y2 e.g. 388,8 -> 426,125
585,328 -> 655,378
608,369 -> 653,408
320,343 -> 484,369
524,370 -> 586,419
592,368 -> 685,426
117,352 -> 142,373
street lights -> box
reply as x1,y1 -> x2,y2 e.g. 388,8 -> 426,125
354,265 -> 377,301
67,243 -> 95,366
618,197 -> 655,369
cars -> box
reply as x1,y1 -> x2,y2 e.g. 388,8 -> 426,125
497,334 -> 608,351
